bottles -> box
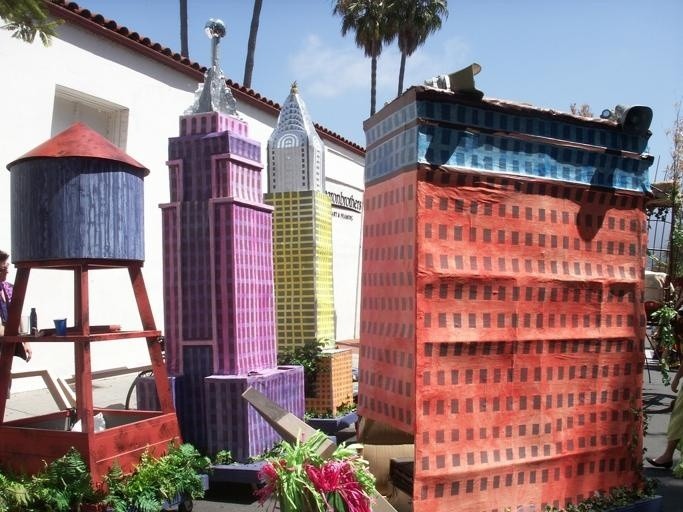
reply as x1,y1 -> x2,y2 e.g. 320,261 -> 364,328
29,307 -> 38,337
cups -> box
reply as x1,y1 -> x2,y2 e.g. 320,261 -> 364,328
53,318 -> 67,337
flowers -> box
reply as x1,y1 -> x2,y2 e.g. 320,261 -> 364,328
253,427 -> 376,512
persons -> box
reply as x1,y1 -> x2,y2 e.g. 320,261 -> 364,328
0,248 -> 31,401
645,285 -> 682,469
